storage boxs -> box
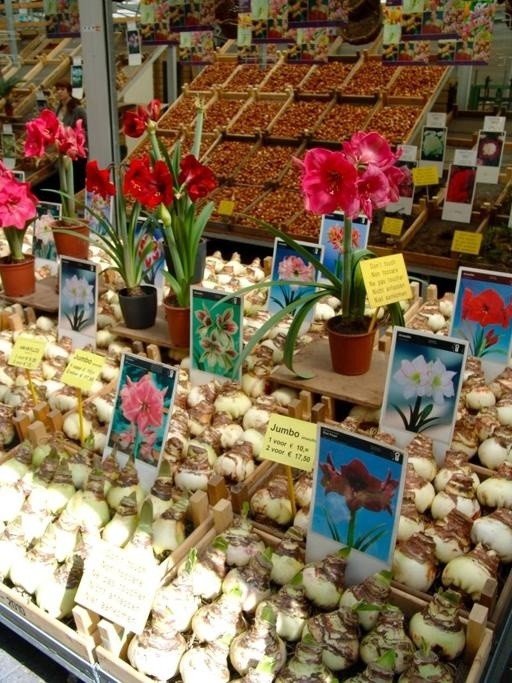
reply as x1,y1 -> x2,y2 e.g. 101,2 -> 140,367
295,54 -> 363,95
291,35 -> 339,56
311,92 -> 382,142
261,60 -> 315,93
223,63 -> 281,94
437,163 -> 510,213
64,349 -> 304,497
221,39 -> 245,58
441,112 -> 497,141
187,92 -> 254,134
387,63 -> 451,99
228,93 -> 291,135
167,129 -> 222,166
312,333 -> 512,477
0,306 -> 162,456
2,425 -> 211,662
94,500 -> 492,683
466,215 -> 512,268
109,221 -> 273,318
238,408 -> 512,623
362,97 -> 431,143
411,126 -> 475,166
370,196 -> 426,247
384,285 -> 511,362
190,60 -> 240,93
267,92 -> 337,137
122,130 -> 178,168
201,185 -> 320,241
252,262 -> 420,337
201,134 -> 261,182
234,137 -> 304,185
155,91 -> 215,132
342,52 -> 406,96
467,132 -> 511,171
0,37 -> 80,119
494,112 -> 512,145
279,140 -> 346,190
404,205 -> 489,262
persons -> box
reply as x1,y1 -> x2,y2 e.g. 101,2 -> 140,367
54,80 -> 87,128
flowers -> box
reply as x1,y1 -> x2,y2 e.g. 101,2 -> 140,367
448,170 -> 474,204
239,0 -> 353,57
64,276 -> 96,329
89,192 -> 111,233
136,0 -> 241,58
205,131 -> 427,378
269,255 -> 317,315
383,0 -> 494,64
118,370 -> 170,459
123,98 -> 213,308
26,107 -> 85,225
127,33 -> 138,48
194,301 -> 238,376
387,356 -> 458,432
396,165 -> 415,197
134,229 -> 159,281
477,136 -> 502,167
319,453 -> 398,553
70,68 -> 84,84
37,161 -> 169,295
324,223 -> 359,278
422,131 -> 444,161
34,210 -> 59,261
463,287 -> 511,357
41,0 -> 82,37
1,167 -> 38,259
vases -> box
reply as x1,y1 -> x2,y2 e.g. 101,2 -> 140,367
50,217 -> 91,261
326,314 -> 379,376
164,292 -> 202,346
163,233 -> 207,284
118,284 -> 157,328
1,254 -> 39,297
